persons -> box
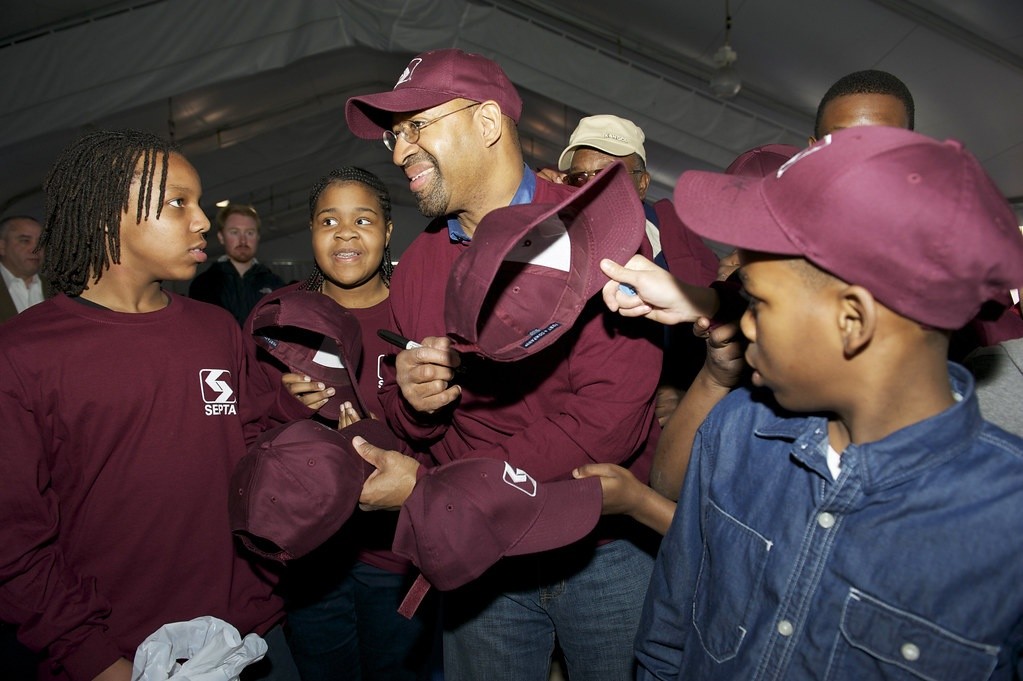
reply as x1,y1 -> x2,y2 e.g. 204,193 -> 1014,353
2,133 -> 308,681
600,70 -> 916,341
344,47 -> 667,681
242,166 -> 445,681
571,338 -> 1022,538
0,212 -> 58,326
645,144 -> 808,502
630,123 -> 1023,681
535,112 -> 721,431
191,199 -> 288,332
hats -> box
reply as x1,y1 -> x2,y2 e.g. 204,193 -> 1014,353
725,143 -> 803,178
393,457 -> 603,621
229,417 -> 406,561
344,47 -> 523,140
250,287 -> 371,423
559,114 -> 647,171
445,161 -> 645,363
672,127 -> 1023,347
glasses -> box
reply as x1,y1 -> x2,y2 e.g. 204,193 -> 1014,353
382,101 -> 483,152
562,168 -> 645,192
